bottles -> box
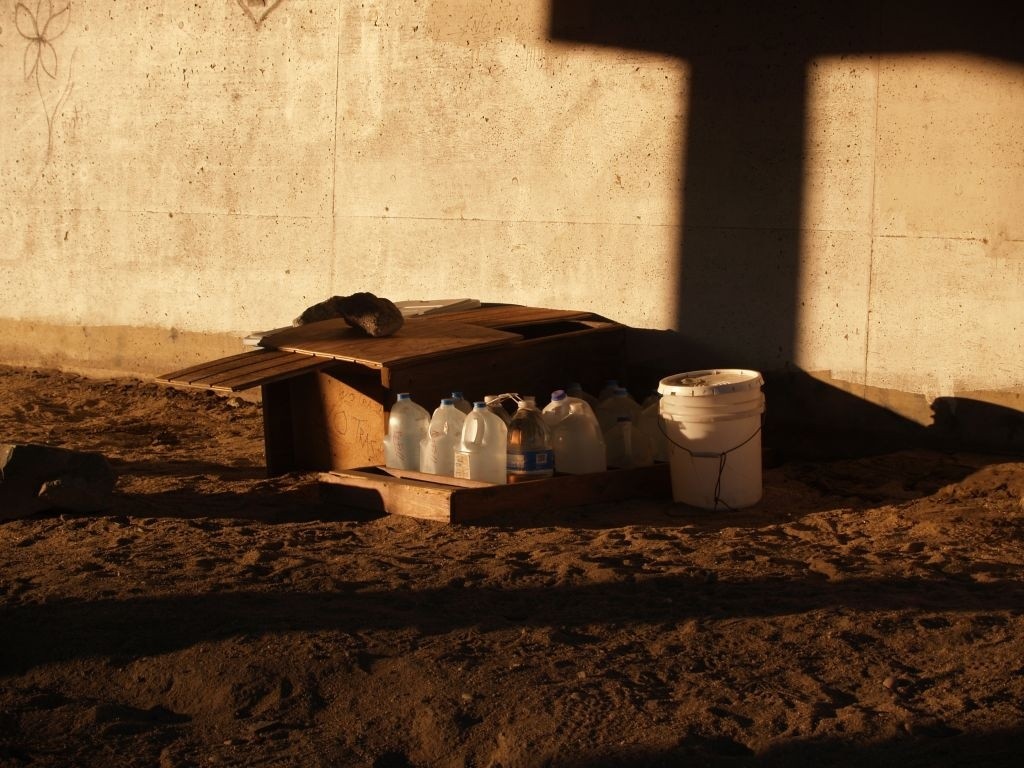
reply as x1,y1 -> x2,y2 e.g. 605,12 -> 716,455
386,380 -> 666,486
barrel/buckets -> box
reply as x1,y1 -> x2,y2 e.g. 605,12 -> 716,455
657,368 -> 766,511
